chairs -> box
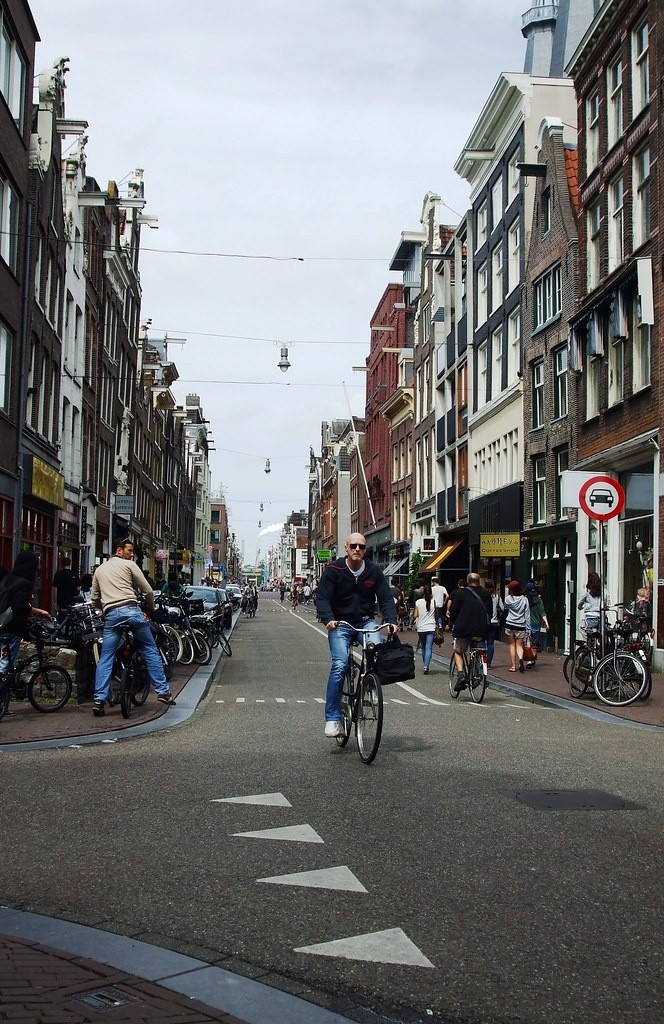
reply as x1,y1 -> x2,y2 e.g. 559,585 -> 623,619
204,593 -> 216,603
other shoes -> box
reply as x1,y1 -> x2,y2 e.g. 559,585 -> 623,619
496,605 -> 506,626
519,659 -> 524,673
91,700 -> 106,716
487,664 -> 491,670
509,667 -> 516,672
454,676 -> 467,691
156,693 -> 178,707
423,668 -> 429,675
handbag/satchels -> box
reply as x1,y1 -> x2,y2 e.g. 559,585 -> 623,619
523,636 -> 537,661
370,626 -> 415,685
433,626 -> 444,644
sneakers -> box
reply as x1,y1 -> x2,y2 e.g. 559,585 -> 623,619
367,690 -> 379,703
323,720 -> 346,738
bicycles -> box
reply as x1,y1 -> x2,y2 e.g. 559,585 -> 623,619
280,590 -> 285,602
327,621 -> 394,764
448,625 -> 490,703
0,588 -> 232,721
244,593 -> 256,618
291,596 -> 298,610
563,609 -> 652,706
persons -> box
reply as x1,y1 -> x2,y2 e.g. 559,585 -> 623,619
387,579 -> 409,632
182,578 -> 190,586
316,533 -> 400,735
578,572 -> 615,647
290,582 -> 317,606
442,573 -> 505,692
51,556 -> 82,626
91,538 -> 177,715
140,568 -> 185,594
413,584 -> 438,673
500,575 -> 550,673
241,581 -> 260,616
265,579 -> 291,592
0,550 -> 54,716
199,577 -> 219,588
413,575 -> 449,631
79,564 -> 100,602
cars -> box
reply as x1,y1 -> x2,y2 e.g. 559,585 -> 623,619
225,584 -> 243,607
181,586 -> 233,630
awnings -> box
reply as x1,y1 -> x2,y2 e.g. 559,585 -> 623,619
417,538 -> 464,572
385,556 -> 409,576
382,559 -> 399,574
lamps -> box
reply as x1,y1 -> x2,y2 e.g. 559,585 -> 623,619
259,502 -> 264,512
459,486 -> 492,496
258,520 -> 261,528
264,458 -> 271,474
277,347 -> 291,373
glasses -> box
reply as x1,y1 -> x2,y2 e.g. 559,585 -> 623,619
348,544 -> 369,550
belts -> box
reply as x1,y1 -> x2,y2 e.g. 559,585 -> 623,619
105,603 -> 137,614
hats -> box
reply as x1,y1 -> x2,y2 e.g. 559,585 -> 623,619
508,581 -> 520,595
525,583 -> 538,595
